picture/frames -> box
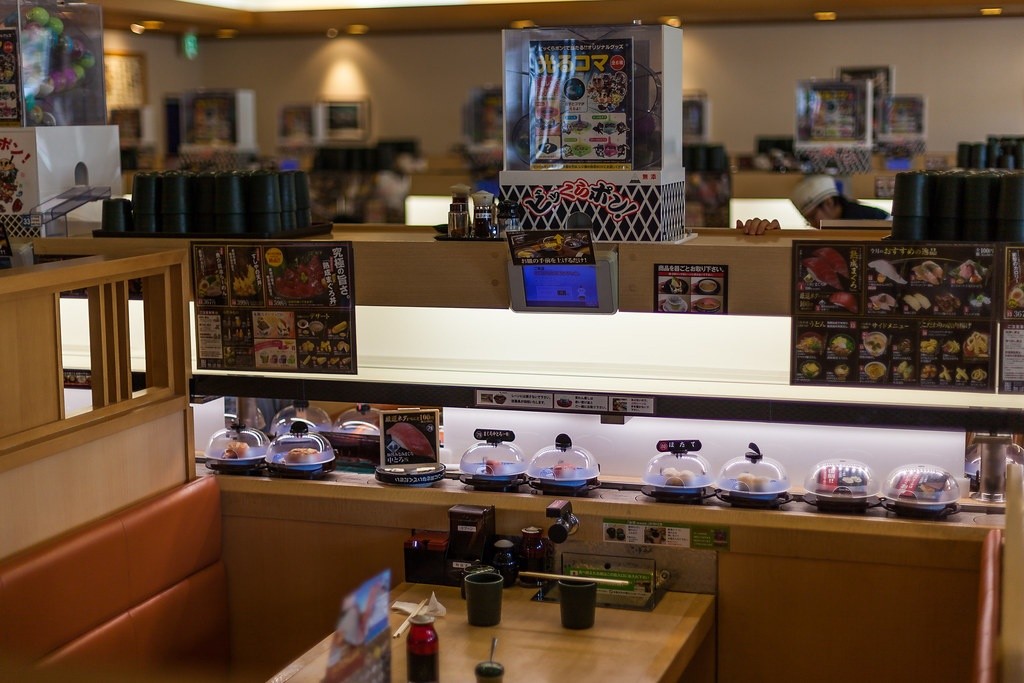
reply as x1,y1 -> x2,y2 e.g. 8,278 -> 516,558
795,64 -> 931,152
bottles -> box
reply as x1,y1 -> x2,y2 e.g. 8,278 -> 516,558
449,184 -> 522,239
493,524 -> 548,587
406,614 -> 441,683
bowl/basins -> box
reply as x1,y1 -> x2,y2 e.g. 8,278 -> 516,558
864,362 -> 886,380
863,331 -> 887,357
830,334 -> 856,358
799,360 -> 822,379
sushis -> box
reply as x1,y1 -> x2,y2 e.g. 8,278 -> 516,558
969,295 -> 992,314
386,422 -> 435,458
805,248 -> 943,311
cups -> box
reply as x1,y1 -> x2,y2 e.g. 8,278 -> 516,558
890,131 -> 1024,244
105,166 -> 310,236
464,572 -> 505,625
558,579 -> 597,629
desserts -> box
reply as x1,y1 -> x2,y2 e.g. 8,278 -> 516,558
737,472 -> 770,492
486,459 -> 503,474
227,441 -> 249,458
662,467 -> 695,486
552,462 -> 574,478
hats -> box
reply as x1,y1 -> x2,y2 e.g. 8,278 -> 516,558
788,174 -> 839,217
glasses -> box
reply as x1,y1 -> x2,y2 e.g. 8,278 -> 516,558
805,202 -> 824,226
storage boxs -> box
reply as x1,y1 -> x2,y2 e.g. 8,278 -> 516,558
500,24 -> 682,171
0,0 -> 109,128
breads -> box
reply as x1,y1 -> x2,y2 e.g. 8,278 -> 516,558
287,448 -> 320,463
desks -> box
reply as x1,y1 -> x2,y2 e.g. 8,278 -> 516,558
267,580 -> 717,683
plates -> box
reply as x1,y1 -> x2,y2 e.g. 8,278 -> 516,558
204,421 -> 958,512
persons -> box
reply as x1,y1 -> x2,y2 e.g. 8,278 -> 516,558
735,175 -> 891,235
330,152 -> 417,221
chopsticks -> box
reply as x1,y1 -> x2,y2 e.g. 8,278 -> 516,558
519,570 -> 628,588
394,599 -> 427,638
